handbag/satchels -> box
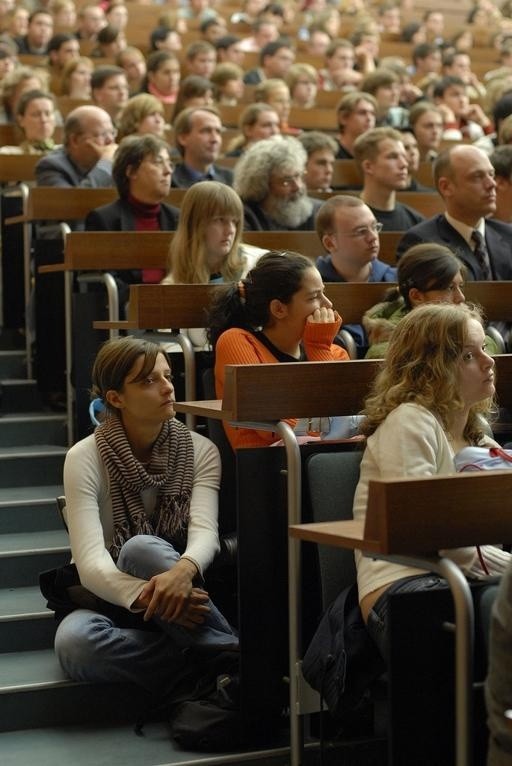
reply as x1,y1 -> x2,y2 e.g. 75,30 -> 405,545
170,699 -> 278,749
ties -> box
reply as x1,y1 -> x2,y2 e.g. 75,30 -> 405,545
471,231 -> 491,280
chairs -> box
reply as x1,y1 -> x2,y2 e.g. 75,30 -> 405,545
308,450 -> 366,615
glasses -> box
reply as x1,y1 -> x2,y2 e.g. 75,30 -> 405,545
265,169 -> 310,185
129,156 -> 178,172
75,128 -> 121,138
326,223 -> 385,235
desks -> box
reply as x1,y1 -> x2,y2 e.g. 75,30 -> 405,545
172,353 -> 512,764
293,467 -> 512,766
88,278 -> 488,432
36,226 -> 418,348
0,182 -> 512,381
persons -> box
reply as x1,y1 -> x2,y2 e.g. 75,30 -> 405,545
76,0 -> 130,63
182,39 -> 217,78
208,61 -> 243,107
207,248 -> 352,451
16,9 -> 63,55
153,181 -> 273,347
297,130 -> 338,195
282,62 -> 319,108
57,63 -> 92,100
42,0 -> 77,32
354,126 -> 424,230
80,136 -> 179,285
359,68 -> 408,128
172,105 -> 234,185
0,45 -> 16,78
1,6 -> 30,55
363,243 -> 504,362
1,90 -> 60,153
112,47 -> 145,93
225,103 -> 281,156
243,41 -> 295,84
140,49 -> 182,117
406,101 -> 442,186
490,144 -> 511,223
141,1 -> 318,47
335,91 -> 379,160
115,93 -> 166,138
396,146 -> 511,282
175,74 -> 217,106
53,338 -> 240,719
353,302 -> 510,685
0,64 -> 50,125
319,1 -> 470,90
251,78 -> 301,133
433,77 -> 496,143
34,106 -> 117,223
87,64 -> 131,124
312,195 -> 398,283
232,134 -> 315,230
48,34 -> 81,76
470,0 -> 511,144
402,130 -> 437,192
